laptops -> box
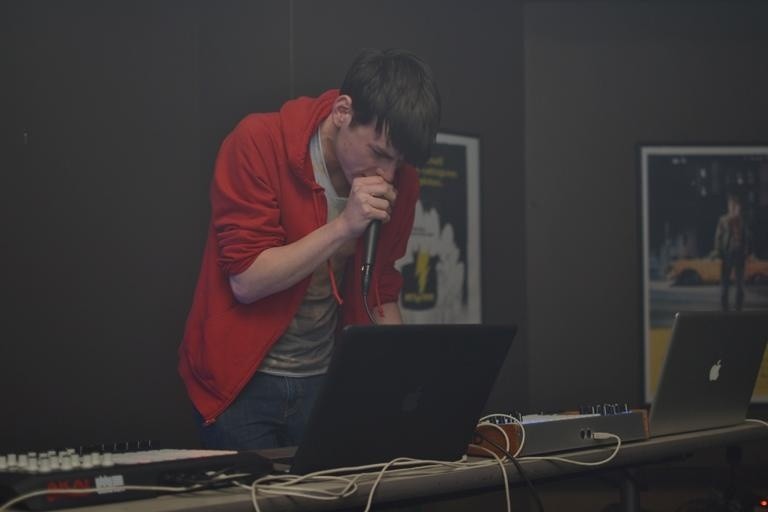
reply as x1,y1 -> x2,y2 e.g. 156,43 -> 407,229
646,312 -> 768,437
260,325 -> 515,482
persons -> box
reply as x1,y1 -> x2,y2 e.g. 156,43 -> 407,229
715,200 -> 751,311
177,48 -> 442,481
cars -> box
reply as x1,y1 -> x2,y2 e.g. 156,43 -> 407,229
667,245 -> 767,288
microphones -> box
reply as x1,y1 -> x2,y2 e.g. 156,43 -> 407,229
362,220 -> 381,296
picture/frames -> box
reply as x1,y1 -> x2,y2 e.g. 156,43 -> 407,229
630,137 -> 767,411
350,123 -> 487,335
